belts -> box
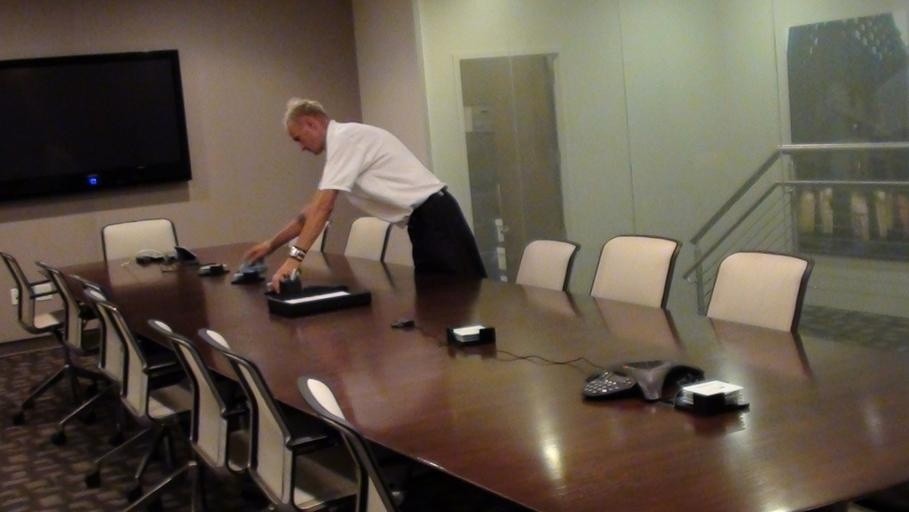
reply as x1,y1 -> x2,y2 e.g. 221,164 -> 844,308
407,187 -> 448,230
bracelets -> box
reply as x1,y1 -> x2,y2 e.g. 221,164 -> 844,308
288,245 -> 306,262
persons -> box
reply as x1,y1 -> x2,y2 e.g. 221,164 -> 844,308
242,96 -> 487,293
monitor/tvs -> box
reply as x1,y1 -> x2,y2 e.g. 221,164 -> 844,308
0,50 -> 193,203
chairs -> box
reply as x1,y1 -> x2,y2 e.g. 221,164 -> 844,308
0,212 -> 908,512
